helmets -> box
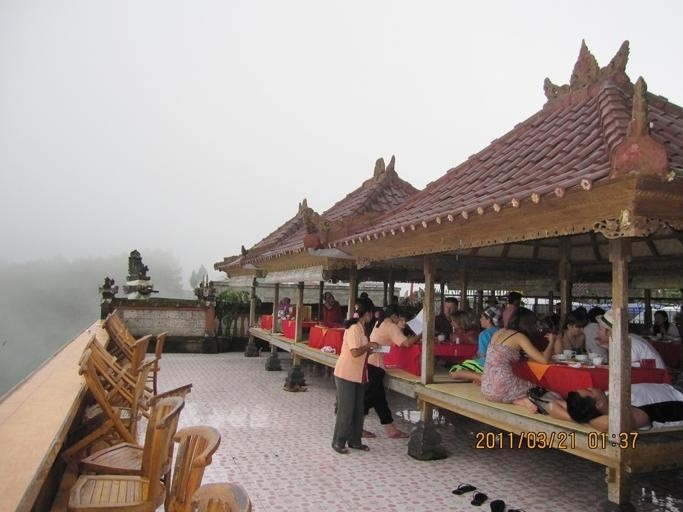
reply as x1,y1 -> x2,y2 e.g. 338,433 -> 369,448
484,306 -> 502,327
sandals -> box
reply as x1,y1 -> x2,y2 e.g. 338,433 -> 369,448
347,442 -> 370,452
361,431 -> 378,438
331,442 -> 349,453
386,430 -> 409,439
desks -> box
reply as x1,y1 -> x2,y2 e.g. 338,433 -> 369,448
259,313 -> 347,355
383,341 -> 478,376
512,335 -> 683,397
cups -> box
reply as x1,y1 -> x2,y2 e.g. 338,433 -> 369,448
551,350 -> 603,367
649,331 -> 682,343
316,319 -> 343,328
434,326 -> 459,344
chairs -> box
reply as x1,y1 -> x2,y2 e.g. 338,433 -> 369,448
60,309 -> 168,474
65,384 -> 253,512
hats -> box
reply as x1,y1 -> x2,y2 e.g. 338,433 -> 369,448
594,308 -> 614,331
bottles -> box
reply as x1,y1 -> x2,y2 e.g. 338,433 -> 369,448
280,305 -> 295,320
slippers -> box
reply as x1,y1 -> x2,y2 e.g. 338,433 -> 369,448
453,482 -> 476,496
471,490 -> 488,506
490,499 -> 525,512
